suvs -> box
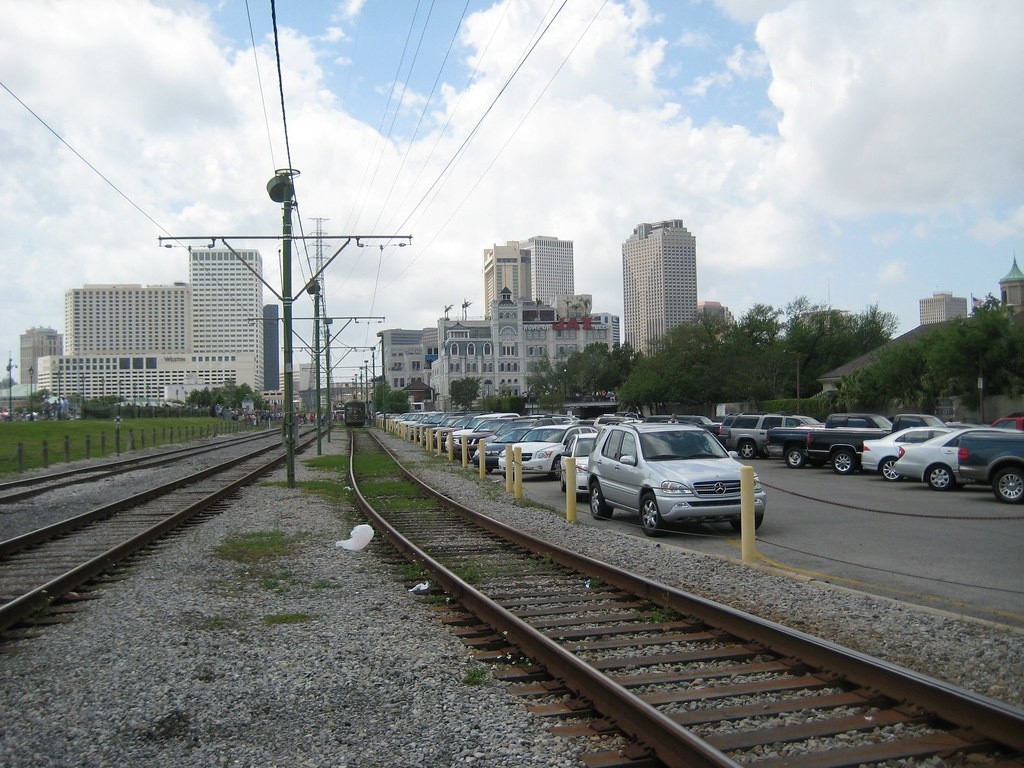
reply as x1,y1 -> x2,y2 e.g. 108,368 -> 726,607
717,412 -> 739,439
725,412 -> 821,460
586,420 -> 768,538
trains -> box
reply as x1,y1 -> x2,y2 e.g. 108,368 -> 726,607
344,398 -> 366,428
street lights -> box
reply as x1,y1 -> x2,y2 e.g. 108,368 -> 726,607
359,366 -> 364,400
784,349 -> 801,414
6,358 -> 18,422
28,366 -> 35,420
158,169 -> 414,488
243,278 -> 387,455
57,370 -> 61,420
364,360 -> 370,422
280,319 -> 377,442
369,346 -> 376,427
355,374 -> 358,398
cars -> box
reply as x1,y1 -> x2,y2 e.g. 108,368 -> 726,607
498,424 -> 599,481
373,410 -> 728,475
945,413 -> 1024,431
893,427 -> 1023,491
860,425 -> 960,482
559,432 -> 599,501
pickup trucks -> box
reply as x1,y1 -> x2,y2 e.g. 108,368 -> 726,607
957,430 -> 1024,504
803,411 -> 948,475
766,412 -> 893,469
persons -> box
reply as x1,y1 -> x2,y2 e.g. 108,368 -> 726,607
668,413 -> 679,424
297,413 -> 343,425
210,403 -> 286,426
126,401 -> 211,417
